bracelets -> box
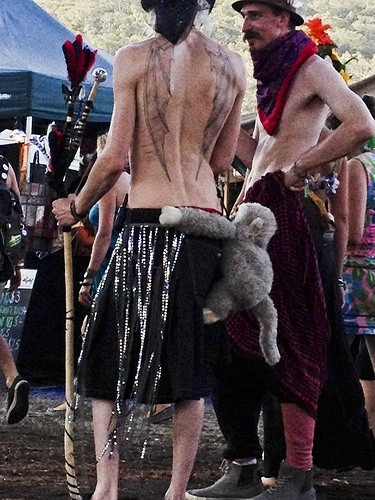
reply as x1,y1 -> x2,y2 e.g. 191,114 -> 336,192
79,281 -> 95,286
70,200 -> 82,221
84,272 -> 96,279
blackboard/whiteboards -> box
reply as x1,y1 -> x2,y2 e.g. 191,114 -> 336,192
0,287 -> 33,363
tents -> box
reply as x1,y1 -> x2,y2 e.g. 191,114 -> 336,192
0,0 -> 114,136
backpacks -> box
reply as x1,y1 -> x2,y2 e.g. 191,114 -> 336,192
0,185 -> 24,283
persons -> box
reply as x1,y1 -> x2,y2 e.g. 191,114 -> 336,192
185,0 -> 375,500
0,154 -> 30,424
52,0 -> 248,500
45,133 -> 173,422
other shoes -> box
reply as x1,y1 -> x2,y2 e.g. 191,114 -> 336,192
149,405 -> 172,423
248,459 -> 316,499
4,374 -> 30,425
184,462 -> 265,499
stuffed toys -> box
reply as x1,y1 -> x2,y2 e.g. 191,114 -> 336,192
160,201 -> 280,367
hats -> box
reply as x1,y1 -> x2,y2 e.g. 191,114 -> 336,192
231,0 -> 304,26
